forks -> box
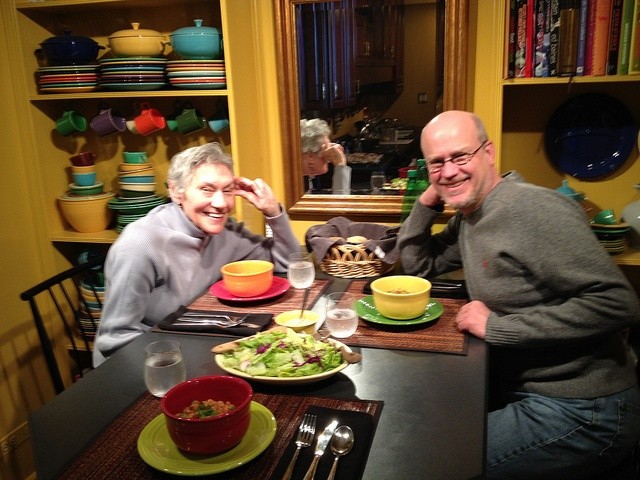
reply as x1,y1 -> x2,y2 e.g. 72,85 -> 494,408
281,413 -> 317,480
173,312 -> 250,333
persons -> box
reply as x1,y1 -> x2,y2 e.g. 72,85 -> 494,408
300,117 -> 351,193
91,141 -> 301,370
397,111 -> 640,479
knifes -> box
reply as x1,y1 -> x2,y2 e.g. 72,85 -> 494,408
305,419 -> 340,479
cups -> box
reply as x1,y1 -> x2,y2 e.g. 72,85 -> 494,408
371,171 -> 387,192
90,108 -> 127,138
70,151 -> 102,165
132,106 -> 167,138
286,251 -> 317,291
52,111 -> 88,135
144,339 -> 188,398
121,148 -> 151,163
170,108 -> 205,136
207,110 -> 237,131
326,291 -> 362,340
593,210 -> 620,224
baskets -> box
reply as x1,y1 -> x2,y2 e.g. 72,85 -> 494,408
320,245 -> 395,278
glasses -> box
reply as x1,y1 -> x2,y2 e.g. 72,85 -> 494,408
425,140 -> 488,174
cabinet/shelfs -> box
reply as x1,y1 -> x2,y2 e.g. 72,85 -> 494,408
1,0 -> 234,351
475,0 -> 640,267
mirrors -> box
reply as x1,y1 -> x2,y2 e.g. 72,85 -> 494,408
272,0 -> 478,223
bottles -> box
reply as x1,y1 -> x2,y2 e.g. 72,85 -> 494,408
417,158 -> 429,194
402,170 -> 418,225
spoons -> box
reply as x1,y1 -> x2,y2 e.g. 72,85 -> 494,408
326,426 -> 356,479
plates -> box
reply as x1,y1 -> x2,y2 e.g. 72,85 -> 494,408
352,296 -> 446,327
137,401 -> 277,480
273,309 -> 320,327
104,196 -> 167,235
34,56 -> 230,92
544,96 -> 640,182
208,276 -> 291,303
213,331 -> 356,383
589,222 -> 632,255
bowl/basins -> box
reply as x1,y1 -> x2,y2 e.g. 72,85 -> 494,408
172,18 -> 223,55
161,375 -> 252,454
68,167 -> 108,199
74,280 -> 108,337
38,26 -> 106,65
108,22 -> 170,56
371,274 -> 432,319
57,201 -> 115,232
220,259 -> 275,296
115,166 -> 159,199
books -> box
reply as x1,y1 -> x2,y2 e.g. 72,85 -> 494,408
504,0 -> 640,79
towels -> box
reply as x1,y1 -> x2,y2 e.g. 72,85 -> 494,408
318,281 -> 470,355
153,277 -> 334,338
158,305 -> 274,336
269,405 -> 372,480
362,276 -> 468,299
50,393 -> 384,480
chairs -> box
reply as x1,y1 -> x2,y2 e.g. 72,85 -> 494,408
19,259 -> 106,398
